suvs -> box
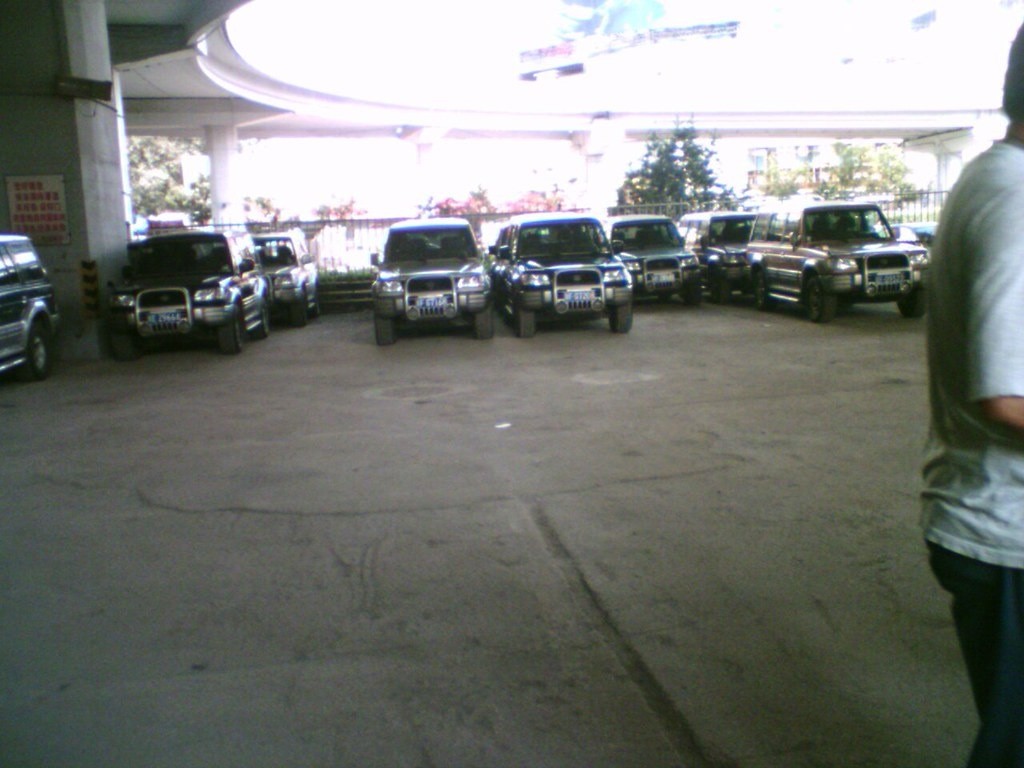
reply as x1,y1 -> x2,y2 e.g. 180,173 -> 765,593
603,216 -> 704,306
676,204 -> 940,324
488,218 -> 634,339
108,226 -> 320,363
369,217 -> 493,348
0,234 -> 59,384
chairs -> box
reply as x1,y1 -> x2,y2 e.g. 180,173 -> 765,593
551,229 -> 579,253
276,246 -> 292,263
835,217 -> 855,236
439,237 -> 466,259
812,218 -> 830,238
722,225 -> 751,244
523,234 -> 549,254
634,230 -> 661,244
403,237 -> 431,260
207,246 -> 232,273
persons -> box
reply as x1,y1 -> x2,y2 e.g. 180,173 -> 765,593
914,19 -> 1024,768
